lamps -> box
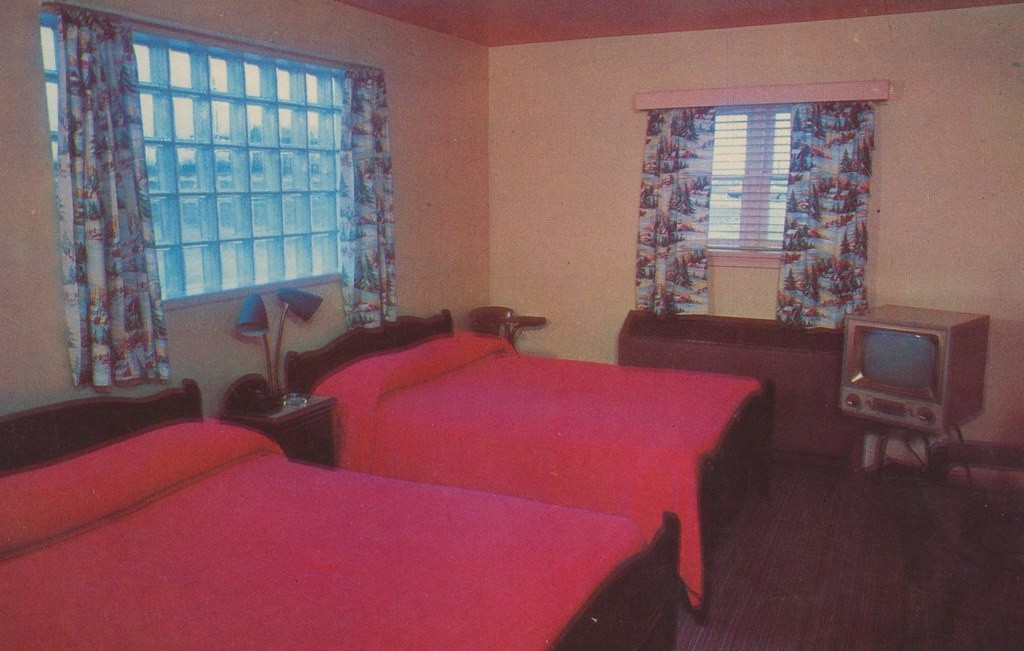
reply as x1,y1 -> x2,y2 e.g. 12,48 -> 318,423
236,286 -> 325,403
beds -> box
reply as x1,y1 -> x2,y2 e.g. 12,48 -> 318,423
0,378 -> 679,651
283,309 -> 777,625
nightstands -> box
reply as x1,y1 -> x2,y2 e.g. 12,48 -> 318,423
467,306 -> 545,355
221,388 -> 337,468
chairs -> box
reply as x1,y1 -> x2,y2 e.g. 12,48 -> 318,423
899,442 -> 1024,651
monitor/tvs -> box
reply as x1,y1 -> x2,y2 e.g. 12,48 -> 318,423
838,305 -> 991,432
